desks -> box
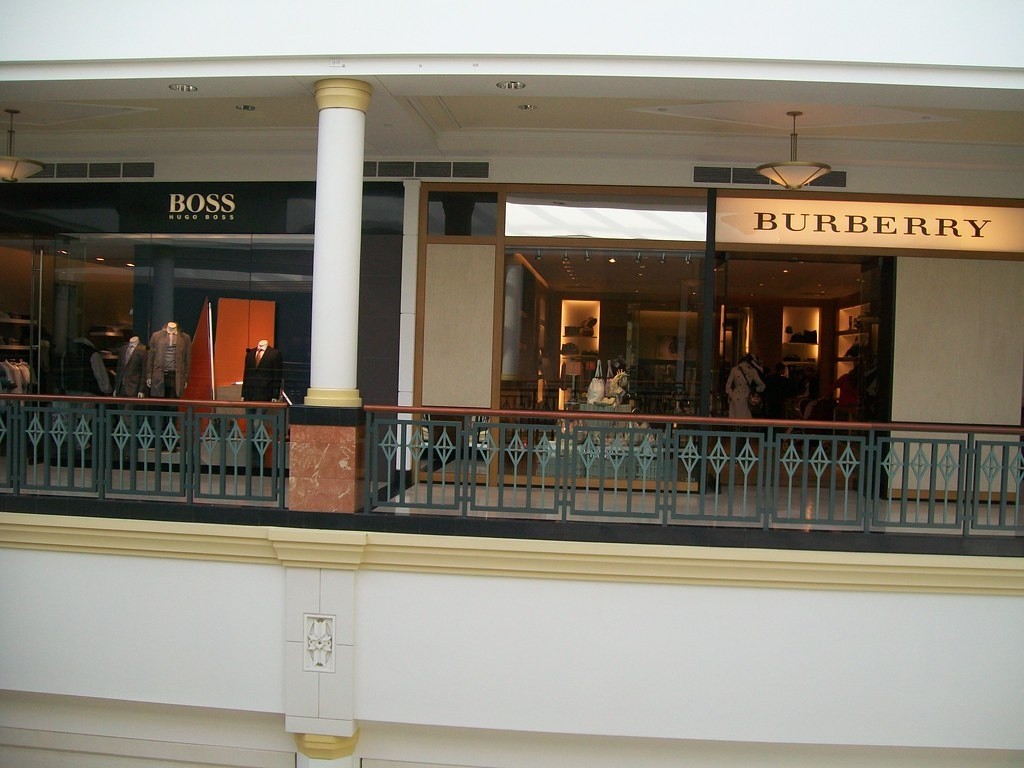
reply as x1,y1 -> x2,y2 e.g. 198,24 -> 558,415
566,401 -> 632,437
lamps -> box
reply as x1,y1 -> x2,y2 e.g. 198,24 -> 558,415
634,251 -> 642,265
683,252 -> 691,264
561,251 -> 568,261
583,250 -> 591,261
607,250 -> 616,263
755,111 -> 830,189
534,248 -> 542,260
60,242 -> 71,255
0,110 -> 44,182
660,253 -> 666,265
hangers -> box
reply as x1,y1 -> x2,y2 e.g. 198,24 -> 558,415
1,353 -> 26,365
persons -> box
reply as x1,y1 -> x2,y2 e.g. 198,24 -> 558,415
240,340 -> 282,465
831,364 -> 860,446
726,345 -> 792,446
61,336 -> 112,455
113,321 -> 192,452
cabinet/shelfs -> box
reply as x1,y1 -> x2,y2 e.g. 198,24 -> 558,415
0,318 -> 38,350
562,334 -> 598,359
87,330 -> 126,361
836,330 -> 869,361
783,342 -> 817,365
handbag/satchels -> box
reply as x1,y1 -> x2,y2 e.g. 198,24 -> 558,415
784,331 -> 817,362
749,393 -> 762,409
587,359 -> 604,404
604,360 -> 620,397
558,415 -> 587,443
631,407 -> 652,445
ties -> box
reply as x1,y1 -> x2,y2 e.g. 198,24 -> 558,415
256,349 -> 262,367
126,344 -> 133,364
